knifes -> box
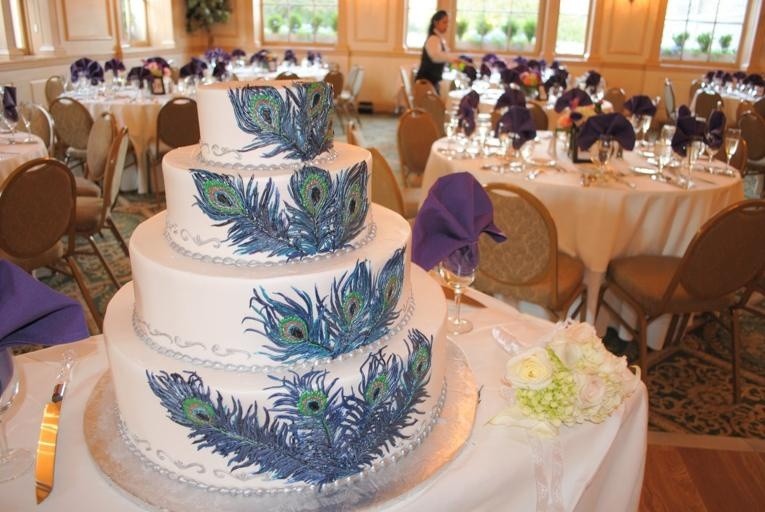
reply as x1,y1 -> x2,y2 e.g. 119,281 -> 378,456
35,350 -> 80,505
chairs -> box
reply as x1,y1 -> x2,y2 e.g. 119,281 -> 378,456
599,200 -> 763,411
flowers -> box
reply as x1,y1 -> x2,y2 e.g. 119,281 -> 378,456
482,319 -> 645,439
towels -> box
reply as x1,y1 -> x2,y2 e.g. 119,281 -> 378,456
2,257 -> 92,393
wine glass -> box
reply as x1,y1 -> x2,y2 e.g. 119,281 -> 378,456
703,67 -> 764,100
443,101 -> 745,188
0,344 -> 37,489
1,97 -> 37,146
66,66 -> 213,102
209,52 -> 330,80
432,58 -> 607,102
437,247 -> 479,339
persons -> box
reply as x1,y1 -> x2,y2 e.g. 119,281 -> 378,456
413,8 -> 465,97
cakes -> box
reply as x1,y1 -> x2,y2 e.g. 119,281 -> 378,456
103,78 -> 447,498
